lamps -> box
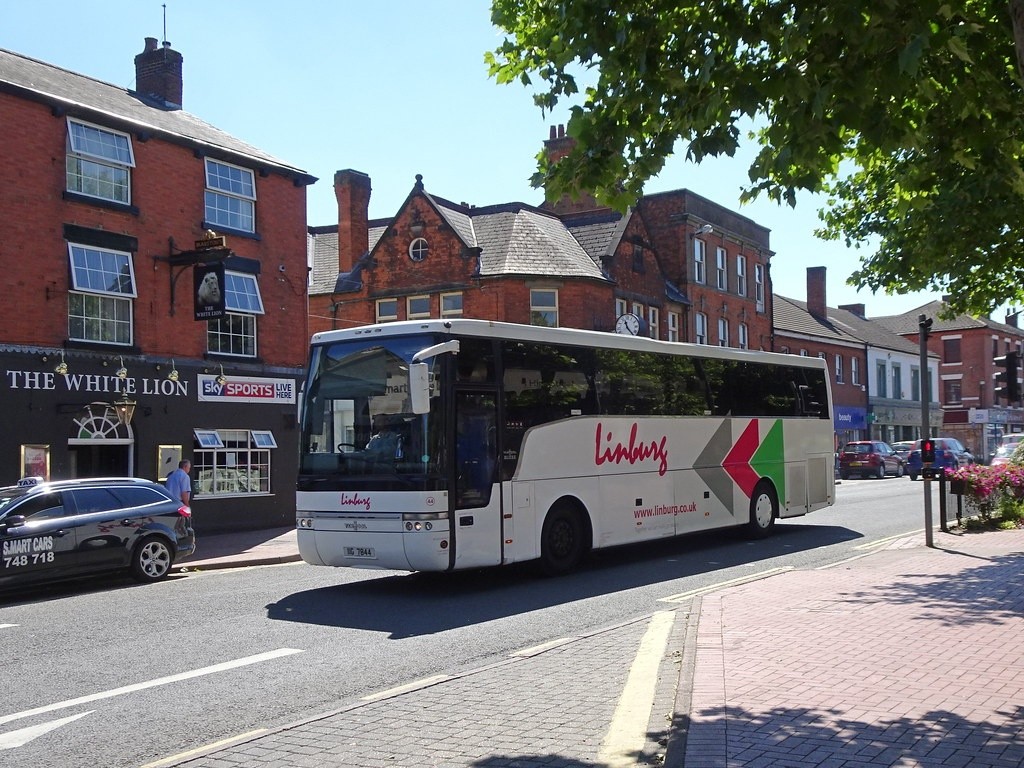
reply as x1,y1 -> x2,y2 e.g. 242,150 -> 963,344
56,386 -> 136,426
103,354 -> 128,379
157,357 -> 178,381
205,363 -> 227,385
43,348 -> 67,375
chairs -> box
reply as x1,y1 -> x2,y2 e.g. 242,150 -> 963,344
371,414 -> 408,438
26,494 -> 60,520
462,414 -> 495,481
506,389 -> 712,418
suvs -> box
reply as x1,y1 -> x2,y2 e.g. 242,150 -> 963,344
906,437 -> 975,481
0,477 -> 196,597
838,439 -> 904,480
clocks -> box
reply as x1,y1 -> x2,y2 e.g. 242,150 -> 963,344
615,314 -> 641,335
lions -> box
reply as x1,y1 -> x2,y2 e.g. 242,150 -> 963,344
197,272 -> 220,305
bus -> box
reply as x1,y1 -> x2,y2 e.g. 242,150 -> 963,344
1001,432 -> 1024,449
291,314 -> 838,578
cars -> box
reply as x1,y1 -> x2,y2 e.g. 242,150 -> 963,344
890,440 -> 915,475
0,475 -> 62,512
989,442 -> 1021,467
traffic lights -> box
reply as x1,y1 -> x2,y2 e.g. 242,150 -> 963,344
992,350 -> 1017,401
921,440 -> 934,462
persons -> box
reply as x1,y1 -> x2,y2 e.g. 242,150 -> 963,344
166,459 -> 191,508
365,414 -> 398,462
